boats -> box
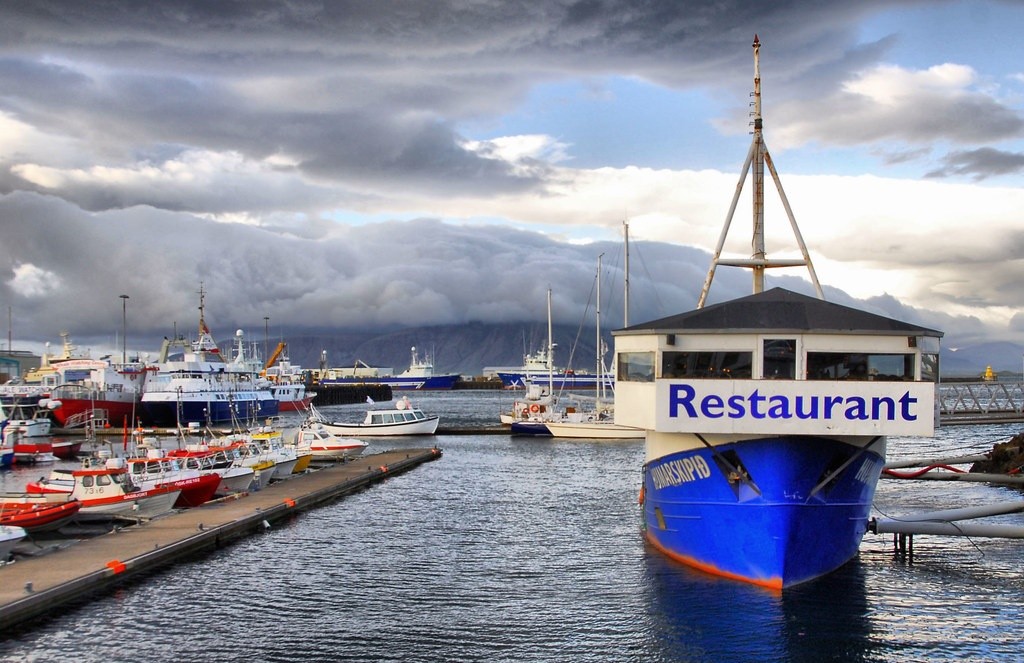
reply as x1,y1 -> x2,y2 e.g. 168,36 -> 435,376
0,287 -> 462,561
266,416 -> 291,427
497,350 -> 615,389
608,35 -> 944,588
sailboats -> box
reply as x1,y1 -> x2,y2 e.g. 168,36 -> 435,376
501,224 -> 667,446
